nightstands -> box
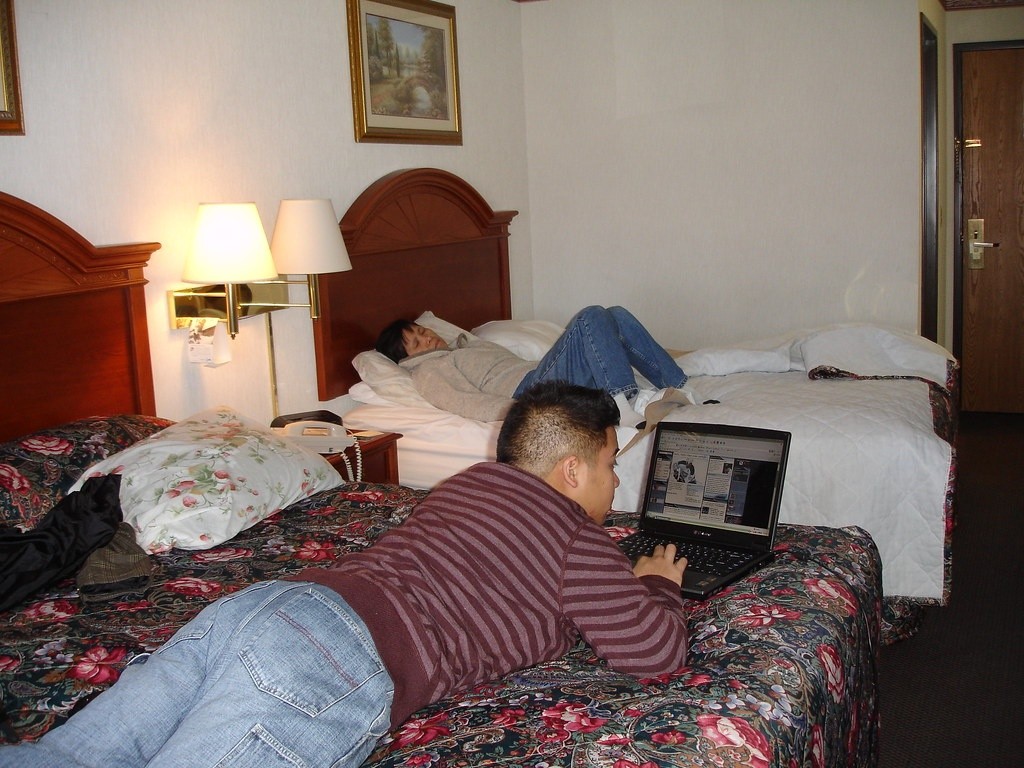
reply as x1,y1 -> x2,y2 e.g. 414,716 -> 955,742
324,428 -> 404,483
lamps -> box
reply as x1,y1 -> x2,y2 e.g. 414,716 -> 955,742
166,200 -> 279,341
240,198 -> 352,322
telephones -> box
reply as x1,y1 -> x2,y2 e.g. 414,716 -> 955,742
269,417 -> 357,455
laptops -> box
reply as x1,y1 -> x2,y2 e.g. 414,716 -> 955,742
614,423 -> 792,603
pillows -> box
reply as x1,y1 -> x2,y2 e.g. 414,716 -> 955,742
470,319 -> 567,361
351,310 -> 482,410
69,406 -> 346,555
0,414 -> 178,525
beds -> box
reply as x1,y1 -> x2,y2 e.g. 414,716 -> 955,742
0,192 -> 884,768
308,166 -> 962,643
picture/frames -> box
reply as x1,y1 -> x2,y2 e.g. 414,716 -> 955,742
346,0 -> 463,147
0,0 -> 25,136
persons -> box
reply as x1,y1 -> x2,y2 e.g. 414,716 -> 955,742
725,465 -> 731,474
1,380 -> 688,768
673,460 -> 696,484
376,304 -> 704,428
